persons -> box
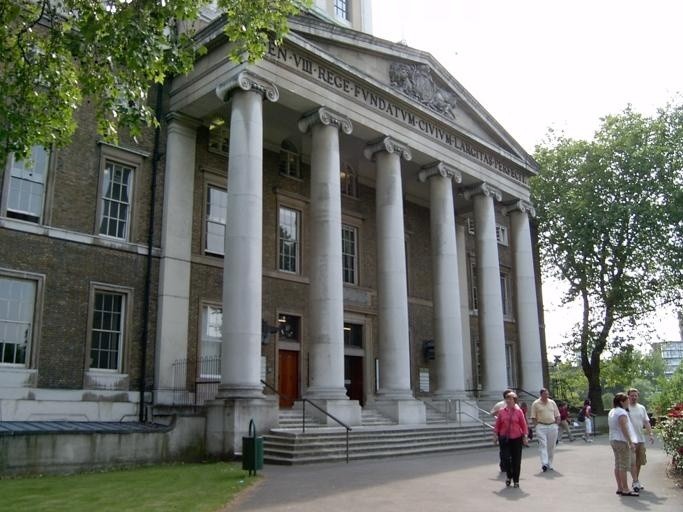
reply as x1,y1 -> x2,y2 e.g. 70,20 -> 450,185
490,389 -> 654,497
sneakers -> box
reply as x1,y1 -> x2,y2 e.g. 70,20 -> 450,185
515,482 -> 519,488
616,482 -> 644,496
506,479 -> 511,486
543,466 -> 548,472
